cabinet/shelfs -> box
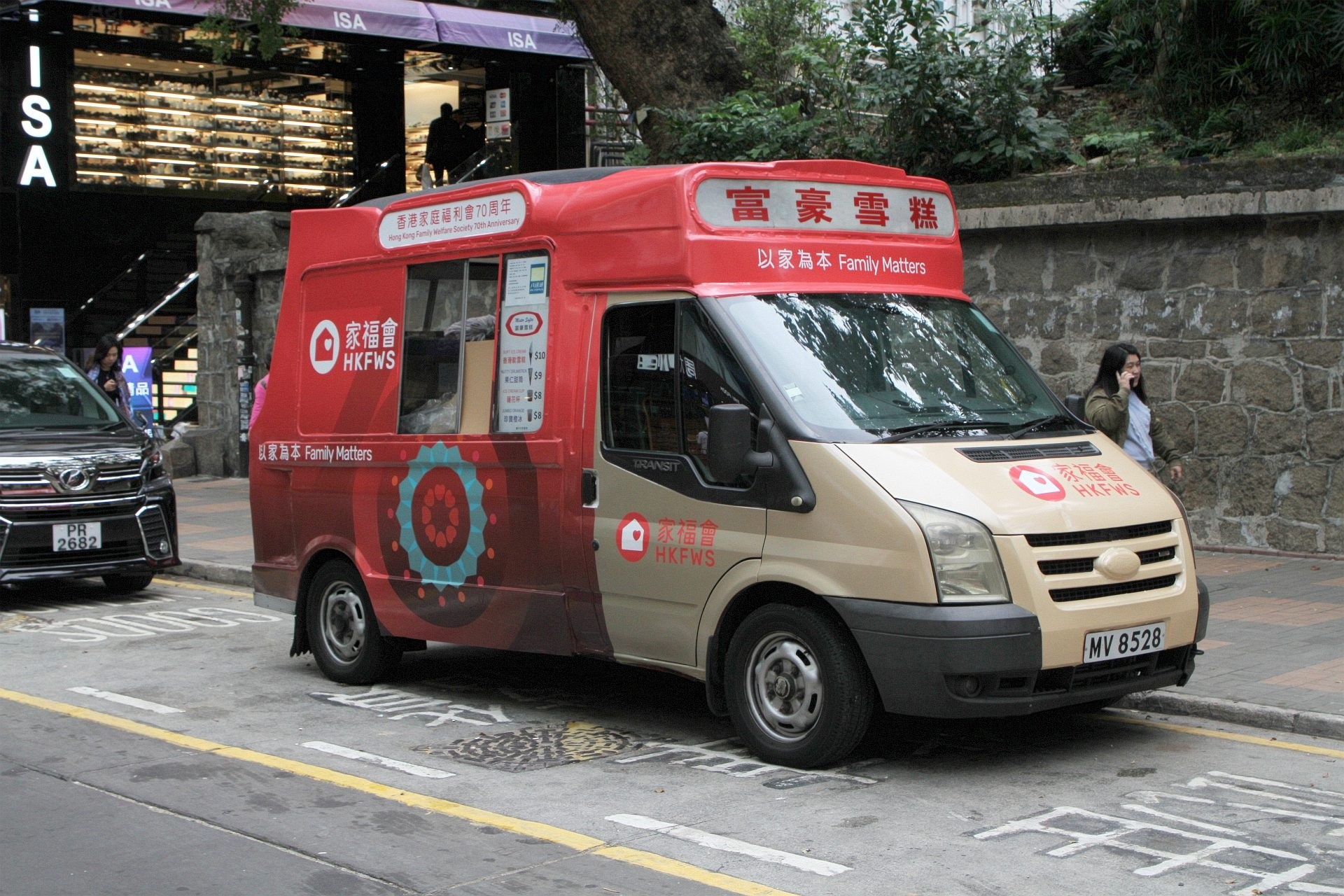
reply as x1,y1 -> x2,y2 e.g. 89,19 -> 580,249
405,124 -> 449,193
74,82 -> 358,199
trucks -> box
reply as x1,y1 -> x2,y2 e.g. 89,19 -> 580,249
249,161 -> 1210,770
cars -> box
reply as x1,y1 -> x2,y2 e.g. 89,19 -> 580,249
0,342 -> 182,594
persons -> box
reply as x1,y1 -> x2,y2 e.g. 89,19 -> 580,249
82,335 -> 134,423
246,349 -> 273,441
1084,344 -> 1183,485
424,103 -> 477,187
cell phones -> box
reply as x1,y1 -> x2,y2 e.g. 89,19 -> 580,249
1117,370 -> 1134,389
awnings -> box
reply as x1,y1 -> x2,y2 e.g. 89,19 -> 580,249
73,0 -> 598,60
